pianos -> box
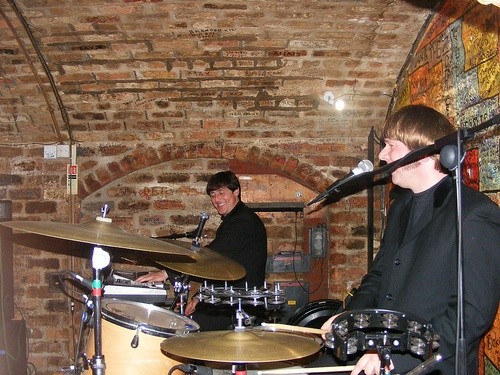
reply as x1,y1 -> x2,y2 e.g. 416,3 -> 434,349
93,269 -> 169,306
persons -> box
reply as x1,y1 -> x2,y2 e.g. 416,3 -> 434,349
134,171 -> 268,333
307,104 -> 500,375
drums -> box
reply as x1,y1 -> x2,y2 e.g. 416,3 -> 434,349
72,297 -> 201,375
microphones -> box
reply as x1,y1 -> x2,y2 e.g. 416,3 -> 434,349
305,159 -> 374,207
195,212 -> 209,242
65,269 -> 104,292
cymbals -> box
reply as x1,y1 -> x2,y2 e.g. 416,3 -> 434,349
193,284 -> 288,311
159,327 -> 327,364
325,307 -> 441,362
145,238 -> 247,281
0,218 -> 197,257
